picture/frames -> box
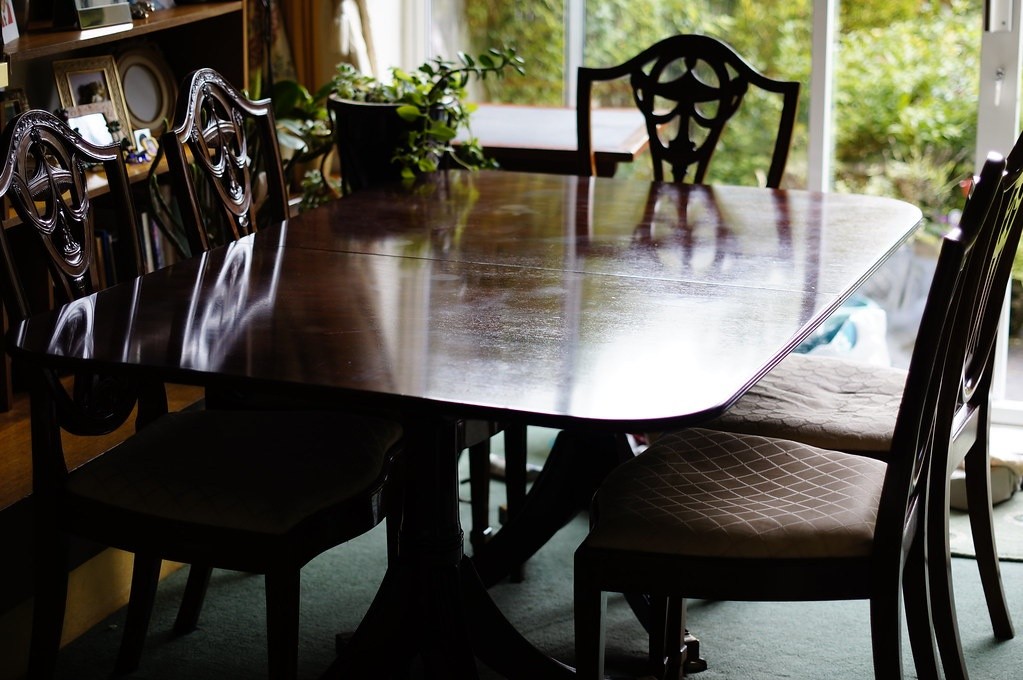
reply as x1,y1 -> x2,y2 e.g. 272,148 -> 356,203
52,53 -> 137,155
0,87 -> 35,171
111,51 -> 179,139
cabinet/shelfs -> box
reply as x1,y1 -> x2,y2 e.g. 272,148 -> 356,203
0,1 -> 253,680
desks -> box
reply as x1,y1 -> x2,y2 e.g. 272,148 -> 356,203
449,103 -> 672,179
0,161 -> 926,677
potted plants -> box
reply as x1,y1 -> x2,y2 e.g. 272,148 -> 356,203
285,42 -> 528,208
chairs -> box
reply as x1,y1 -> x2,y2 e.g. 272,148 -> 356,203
578,35 -> 801,190
0,109 -> 400,680
654,129 -> 1022,680
159,68 -> 527,586
575,130 -> 1007,680
